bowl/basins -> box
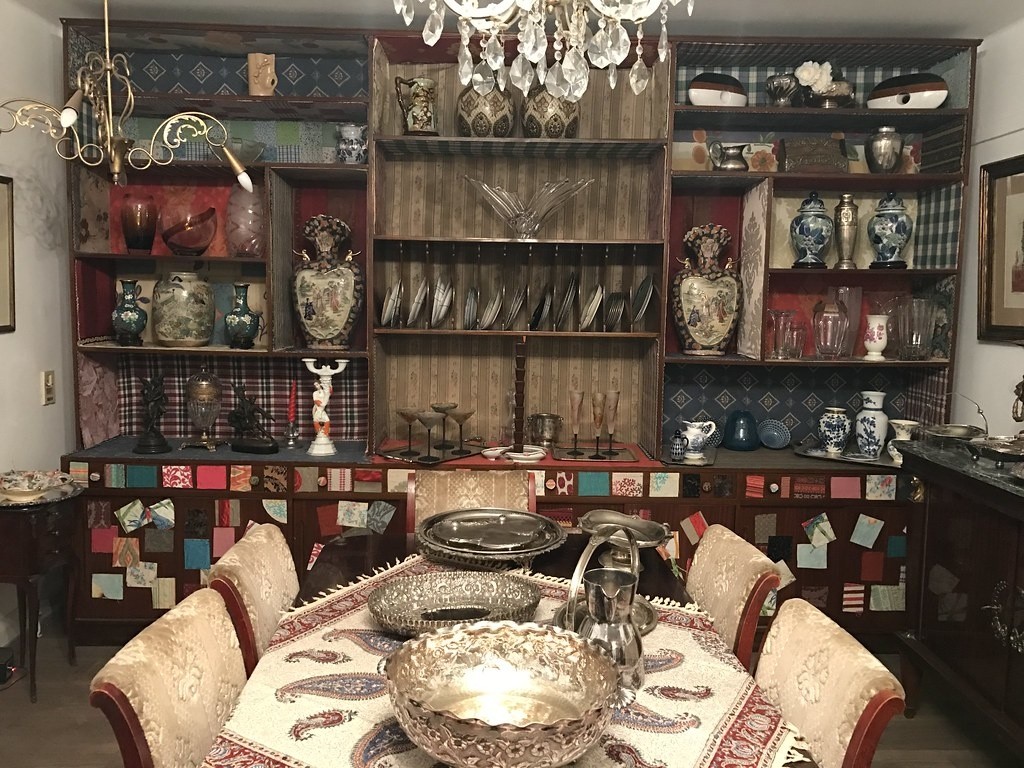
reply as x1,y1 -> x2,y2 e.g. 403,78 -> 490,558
757,418 -> 791,449
383,619 -> 623,768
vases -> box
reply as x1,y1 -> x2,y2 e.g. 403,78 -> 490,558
765,74 -> 799,107
457,68 -> 515,138
290,213 -> 364,350
671,222 -> 743,356
804,63 -> 856,109
887,419 -> 918,462
864,126 -> 905,174
856,391 -> 888,458
817,407 -> 852,454
111,273 -> 260,350
520,76 -> 581,139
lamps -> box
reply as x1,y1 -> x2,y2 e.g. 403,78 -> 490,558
0,0 -> 254,194
393,0 -> 695,103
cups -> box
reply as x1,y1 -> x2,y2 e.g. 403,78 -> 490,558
394,76 -> 440,137
248,52 -> 278,97
766,308 -> 797,360
897,297 -> 938,361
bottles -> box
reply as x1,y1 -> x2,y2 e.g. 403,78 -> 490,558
864,125 -> 905,174
121,193 -> 157,256
670,431 -> 689,463
519,73 -> 582,139
455,69 -> 517,138
789,320 -> 807,360
887,418 -> 919,464
111,280 -> 148,347
819,408 -> 851,454
151,270 -> 215,348
855,391 -> 888,459
223,282 -> 259,349
861,313 -> 889,362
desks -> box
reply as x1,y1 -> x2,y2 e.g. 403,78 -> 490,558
196,530 -> 822,768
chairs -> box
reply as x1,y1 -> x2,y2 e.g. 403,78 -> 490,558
89,522 -> 906,768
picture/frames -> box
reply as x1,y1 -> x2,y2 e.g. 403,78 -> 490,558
977,154 -> 1024,346
0,176 -> 16,334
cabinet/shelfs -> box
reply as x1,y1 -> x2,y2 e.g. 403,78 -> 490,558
0,490 -> 84,703
892,440 -> 1024,768
59,15 -> 983,655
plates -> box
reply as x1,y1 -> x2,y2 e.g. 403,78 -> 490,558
381,271 -> 653,328
0,469 -> 74,502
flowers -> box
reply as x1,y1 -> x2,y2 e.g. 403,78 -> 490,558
793,60 -> 834,94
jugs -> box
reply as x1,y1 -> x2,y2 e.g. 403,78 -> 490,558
709,139 -> 749,171
566,524 -> 647,693
335,121 -> 369,165
681,420 -> 716,452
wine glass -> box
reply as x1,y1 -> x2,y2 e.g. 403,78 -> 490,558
588,392 -> 607,459
399,402 -> 475,462
602,390 -> 621,457
566,389 -> 585,456
